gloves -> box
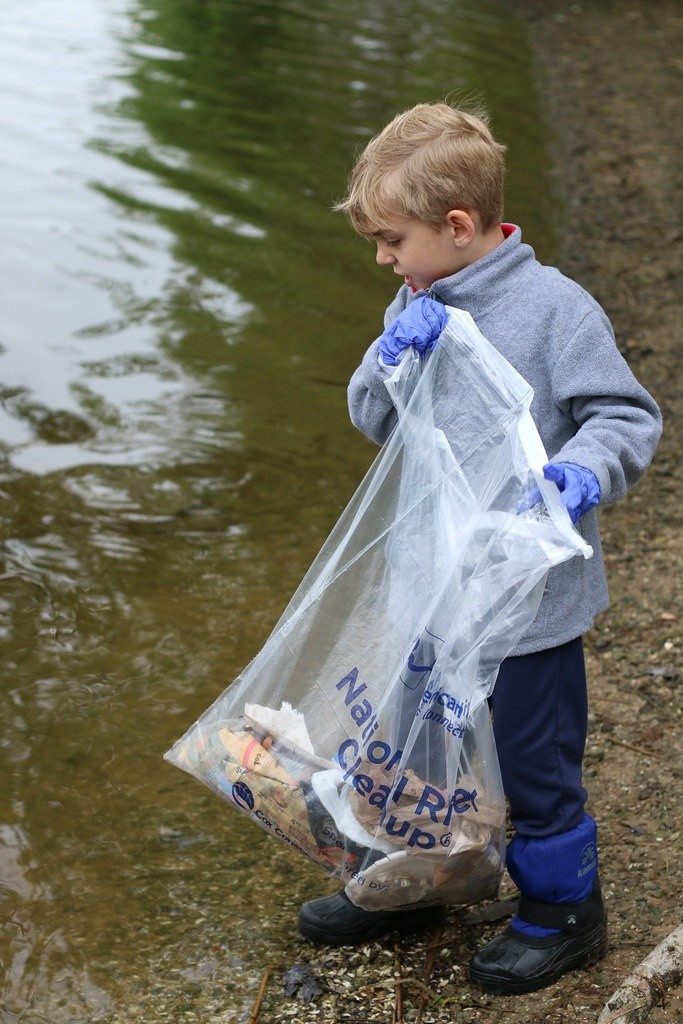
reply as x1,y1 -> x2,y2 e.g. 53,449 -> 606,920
517,462 -> 600,526
378,295 -> 449,365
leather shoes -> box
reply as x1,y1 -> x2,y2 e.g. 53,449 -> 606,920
298,889 -> 446,945
468,870 -> 609,994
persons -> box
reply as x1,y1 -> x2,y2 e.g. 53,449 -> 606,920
295,102 -> 662,995
